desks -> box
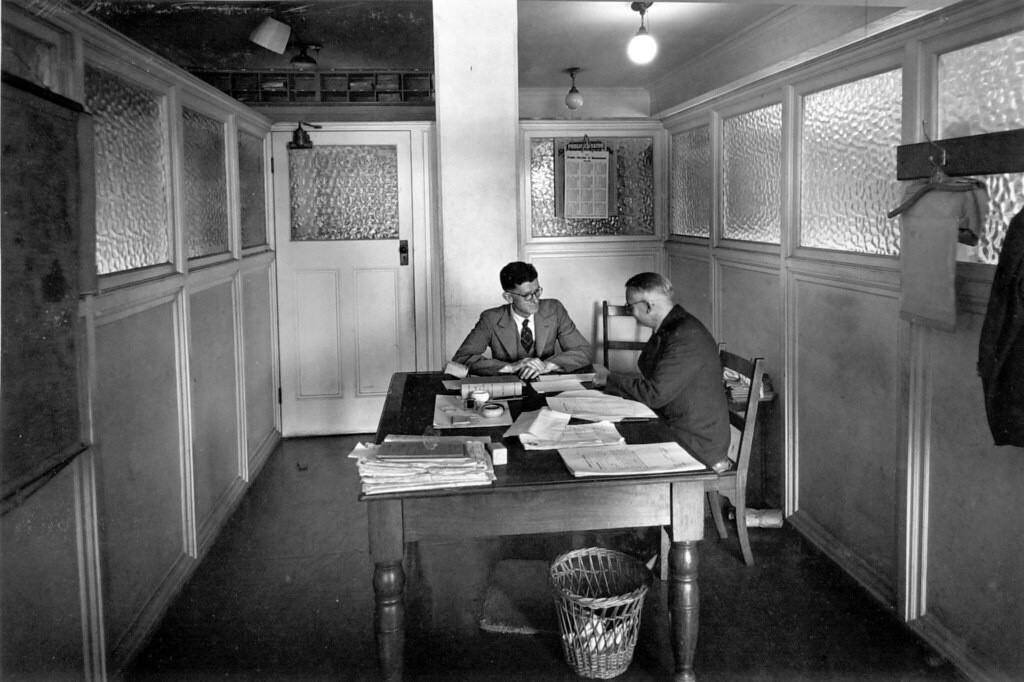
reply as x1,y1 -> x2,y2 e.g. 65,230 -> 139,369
722,377 -> 781,525
356,372 -> 719,682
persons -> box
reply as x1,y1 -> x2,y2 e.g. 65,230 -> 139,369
592,273 -> 732,557
452,262 -> 594,380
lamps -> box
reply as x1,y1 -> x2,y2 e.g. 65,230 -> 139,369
628,2 -> 659,64
563,67 -> 583,108
289,46 -> 317,69
248,12 -> 292,55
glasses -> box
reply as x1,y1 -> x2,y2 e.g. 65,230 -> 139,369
625,300 -> 655,314
506,286 -> 543,301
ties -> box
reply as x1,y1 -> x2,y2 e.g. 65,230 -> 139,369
520,318 -> 533,354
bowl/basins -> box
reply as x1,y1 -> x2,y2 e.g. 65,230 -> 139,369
470,391 -> 489,403
483,405 -> 503,417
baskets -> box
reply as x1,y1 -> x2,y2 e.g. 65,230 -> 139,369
548,547 -> 654,679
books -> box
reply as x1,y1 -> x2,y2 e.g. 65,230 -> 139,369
351,442 -> 496,494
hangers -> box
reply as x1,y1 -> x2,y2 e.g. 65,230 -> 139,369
888,153 -> 981,218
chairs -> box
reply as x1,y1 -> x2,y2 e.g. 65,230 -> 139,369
600,300 -> 652,367
658,342 -> 767,580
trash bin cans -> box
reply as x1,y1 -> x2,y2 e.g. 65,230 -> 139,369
549,546 -> 650,680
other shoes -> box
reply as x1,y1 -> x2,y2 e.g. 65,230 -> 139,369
596,530 -> 655,565
